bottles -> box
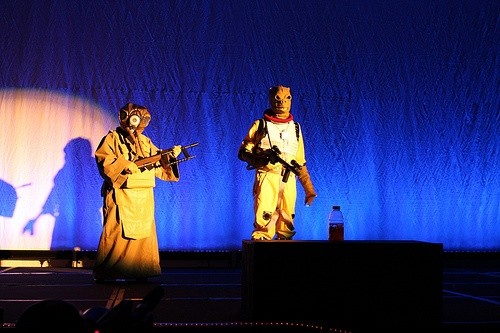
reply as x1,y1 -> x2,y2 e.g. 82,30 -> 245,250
329,206 -> 344,241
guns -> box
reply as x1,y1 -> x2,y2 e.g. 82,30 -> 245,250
256,144 -> 303,183
134,142 -> 197,169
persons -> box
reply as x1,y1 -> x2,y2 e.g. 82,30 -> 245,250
238,85 -> 317,241
93,102 -> 182,283
15,299 -> 154,333
